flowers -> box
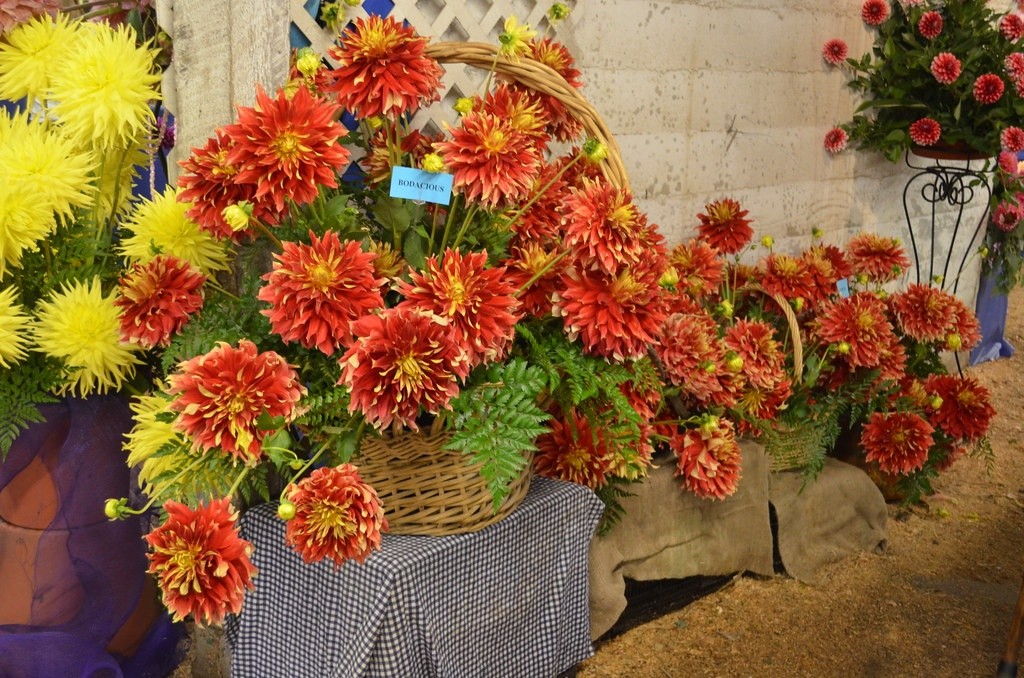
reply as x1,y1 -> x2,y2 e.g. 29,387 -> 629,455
0,0 -> 1023,629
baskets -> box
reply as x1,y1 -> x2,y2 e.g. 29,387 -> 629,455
725,280 -> 832,474
297,418 -> 535,535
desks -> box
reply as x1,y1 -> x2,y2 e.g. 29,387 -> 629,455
234,475 -> 602,677
